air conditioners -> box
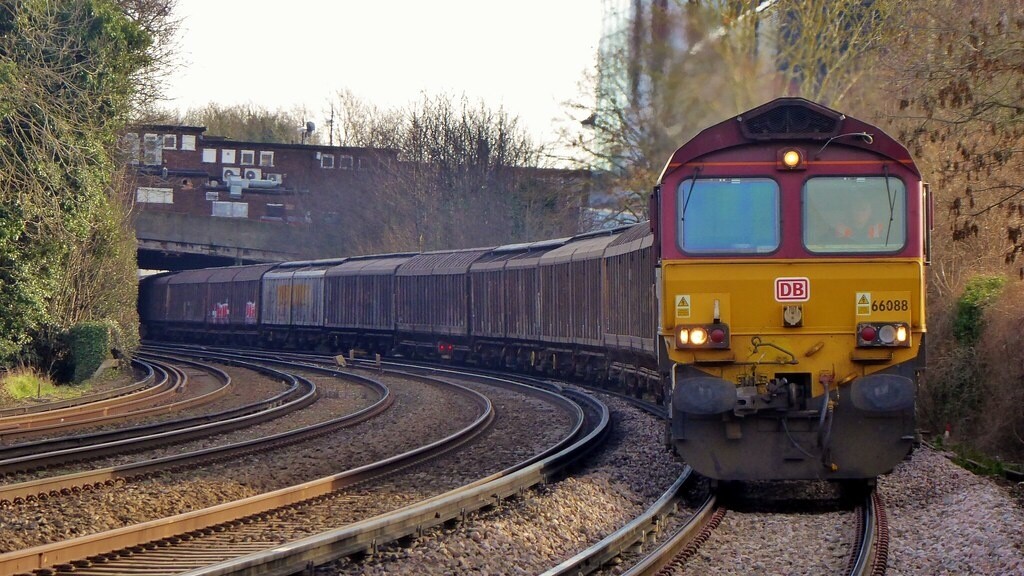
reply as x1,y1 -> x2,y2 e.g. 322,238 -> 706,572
267,174 -> 282,184
144,140 -> 162,166
222,167 -> 240,182
244,168 -> 261,180
121,139 -> 140,165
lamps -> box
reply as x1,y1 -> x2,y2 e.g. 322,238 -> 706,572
311,152 -> 321,160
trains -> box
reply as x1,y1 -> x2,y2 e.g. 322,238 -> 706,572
140,98 -> 937,504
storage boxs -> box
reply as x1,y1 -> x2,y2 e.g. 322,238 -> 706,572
212,202 -> 248,218
